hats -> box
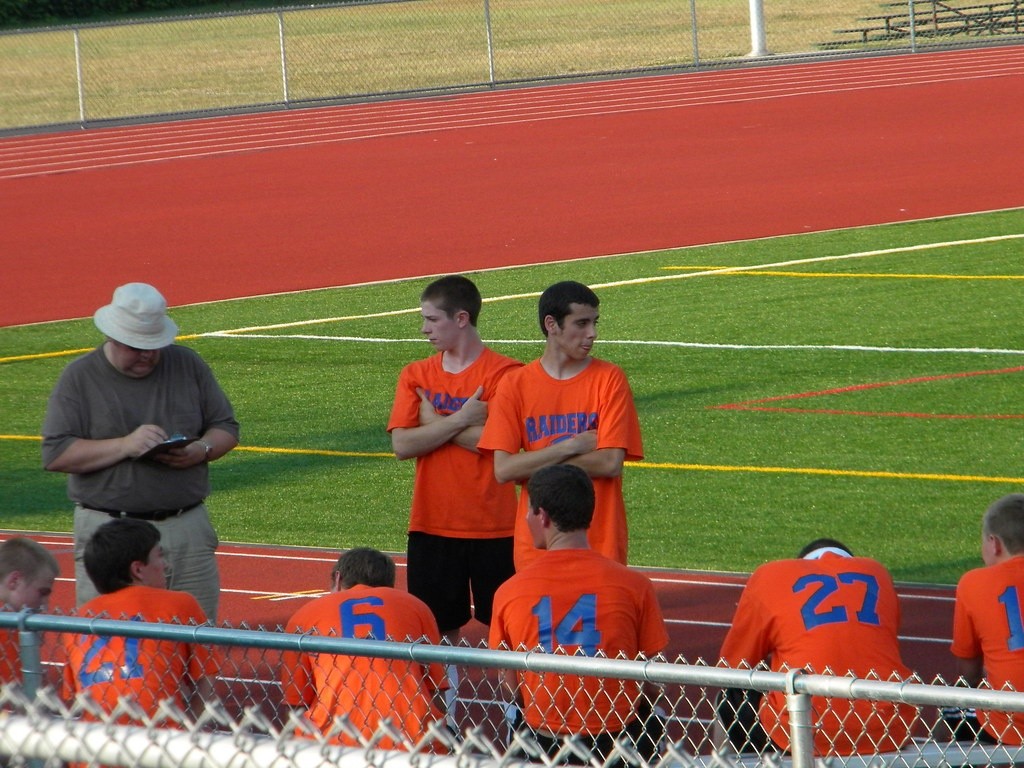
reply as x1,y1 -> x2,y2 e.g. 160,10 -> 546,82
94,282 -> 177,350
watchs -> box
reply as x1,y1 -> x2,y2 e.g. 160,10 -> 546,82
196,438 -> 214,463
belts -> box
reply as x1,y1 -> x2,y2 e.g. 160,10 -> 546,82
82,500 -> 205,520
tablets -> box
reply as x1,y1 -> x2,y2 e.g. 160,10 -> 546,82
134,437 -> 202,464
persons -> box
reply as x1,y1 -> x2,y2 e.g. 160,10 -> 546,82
386,275 -> 530,644
488,464 -> 667,767
43,282 -> 239,628
936,494 -> 1023,748
60,519 -> 222,768
475,280 -> 644,570
1,537 -> 59,712
712,538 -> 918,755
280,546 -> 458,754
